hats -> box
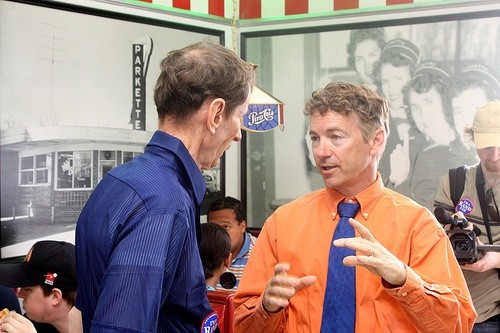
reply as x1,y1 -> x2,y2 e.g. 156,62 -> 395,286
349,26 -> 385,41
411,60 -> 452,84
380,38 -> 423,68
0,240 -> 79,292
472,99 -> 500,150
459,62 -> 500,97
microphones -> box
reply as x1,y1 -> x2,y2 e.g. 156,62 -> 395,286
434,207 -> 453,225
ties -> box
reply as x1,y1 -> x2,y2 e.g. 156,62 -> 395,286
318,202 -> 359,333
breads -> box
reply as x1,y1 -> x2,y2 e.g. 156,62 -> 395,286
0,308 -> 16,319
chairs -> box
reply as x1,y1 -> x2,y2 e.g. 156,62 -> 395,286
205,228 -> 261,333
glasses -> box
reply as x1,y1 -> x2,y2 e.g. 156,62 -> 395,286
486,188 -> 500,222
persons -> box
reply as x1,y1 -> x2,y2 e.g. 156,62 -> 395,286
200,222 -> 232,292
207,196 -> 257,291
75,41 -> 258,333
233,82 -> 477,333
0,240 -> 83,333
433,100 -> 500,280
0,285 -> 22,315
347,28 -> 500,216
